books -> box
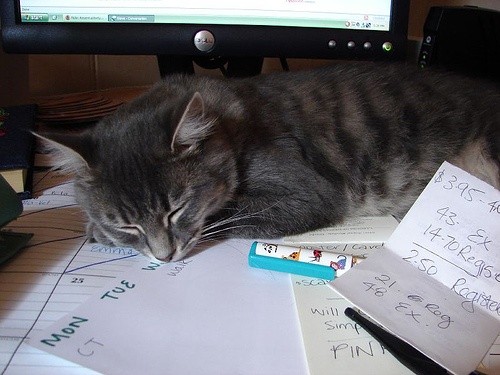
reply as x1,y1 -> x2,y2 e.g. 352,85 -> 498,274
1,105 -> 37,199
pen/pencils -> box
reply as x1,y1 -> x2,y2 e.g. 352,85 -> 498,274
248,241 -> 367,281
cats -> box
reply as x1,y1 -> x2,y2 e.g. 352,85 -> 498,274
20,63 -> 500,265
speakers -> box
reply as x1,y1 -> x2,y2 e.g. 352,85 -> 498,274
417,6 -> 500,71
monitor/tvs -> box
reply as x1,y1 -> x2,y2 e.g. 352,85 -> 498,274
0,0 -> 411,81
